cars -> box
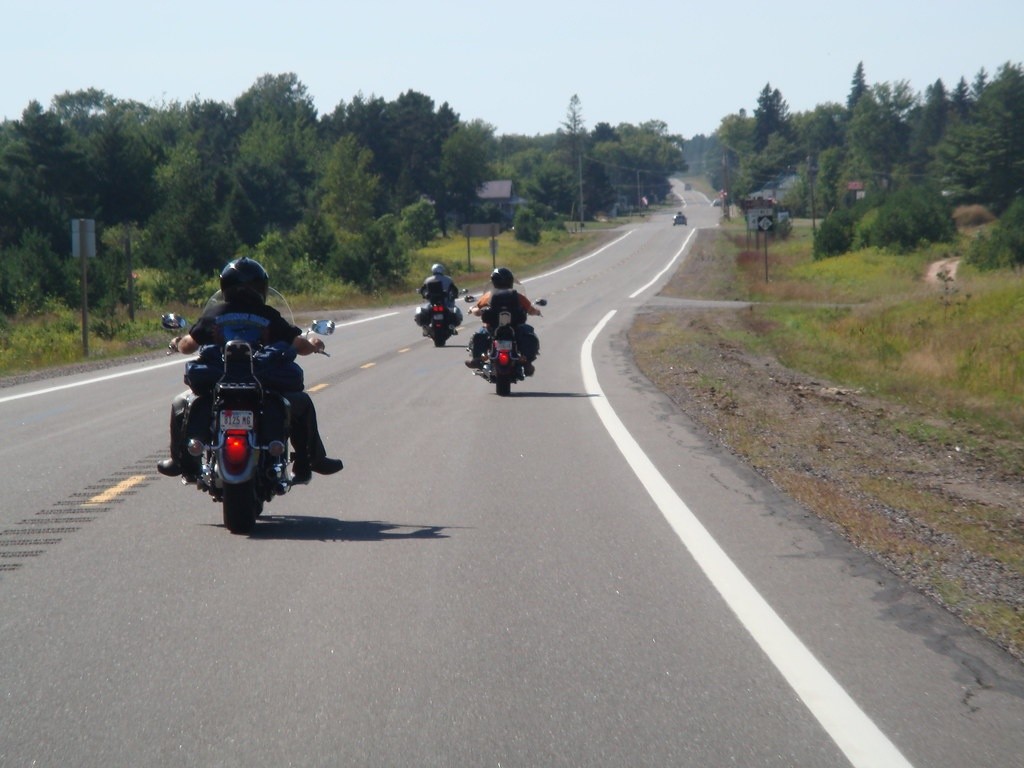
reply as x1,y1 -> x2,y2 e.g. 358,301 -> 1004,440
713,199 -> 721,207
684,183 -> 691,191
673,212 -> 687,225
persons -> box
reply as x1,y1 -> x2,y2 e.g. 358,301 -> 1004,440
465,267 -> 540,377
414,262 -> 459,336
156,255 -> 344,477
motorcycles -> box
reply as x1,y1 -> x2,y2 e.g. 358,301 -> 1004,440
161,287 -> 335,533
465,278 -> 546,395
415,288 -> 467,348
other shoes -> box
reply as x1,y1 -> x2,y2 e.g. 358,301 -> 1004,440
465,360 -> 483,369
524,363 -> 535,376
453,330 -> 459,335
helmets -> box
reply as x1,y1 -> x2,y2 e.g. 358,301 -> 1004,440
219,256 -> 269,304
491,268 -> 514,288
432,264 -> 446,275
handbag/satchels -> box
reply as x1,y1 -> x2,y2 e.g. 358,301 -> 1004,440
184,341 -> 305,396
482,307 -> 527,325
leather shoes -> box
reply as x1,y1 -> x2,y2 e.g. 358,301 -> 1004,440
157,458 -> 202,477
292,457 -> 343,475
